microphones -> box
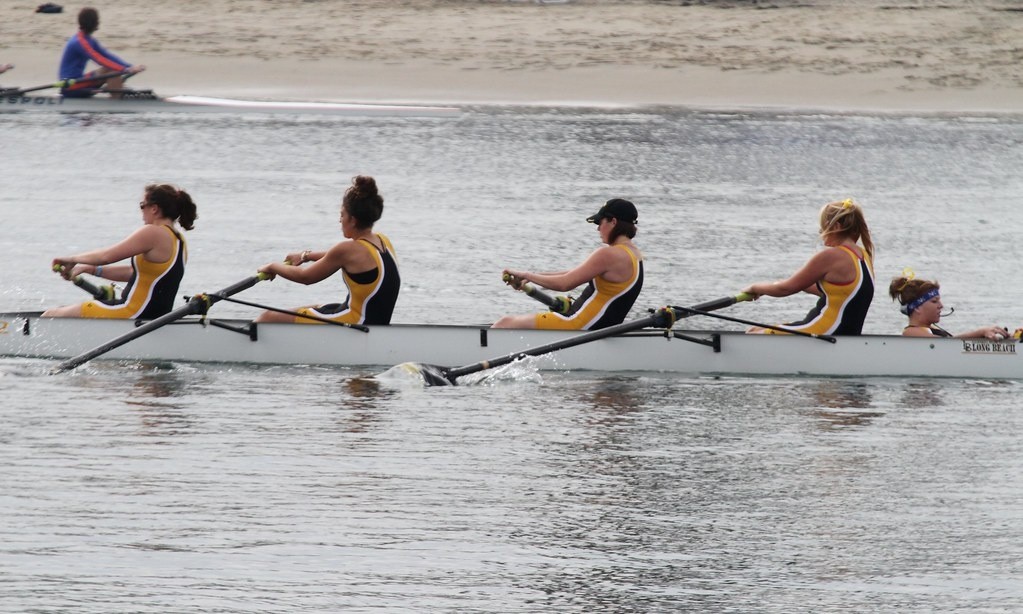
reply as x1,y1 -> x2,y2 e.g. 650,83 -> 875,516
939,307 -> 954,317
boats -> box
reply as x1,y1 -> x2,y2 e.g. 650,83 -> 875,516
1,87 -> 463,117
1,313 -> 1022,379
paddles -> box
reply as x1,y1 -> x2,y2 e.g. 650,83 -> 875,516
417,288 -> 759,387
45,257 -> 308,374
501,270 -> 583,316
0,68 -> 142,97
49,260 -> 122,304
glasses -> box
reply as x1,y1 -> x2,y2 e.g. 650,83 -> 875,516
140,201 -> 156,209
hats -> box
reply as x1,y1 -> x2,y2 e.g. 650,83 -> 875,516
586,199 -> 638,224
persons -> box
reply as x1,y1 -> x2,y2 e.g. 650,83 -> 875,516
40,184 -> 198,321
253,175 -> 401,324
58,7 -> 145,99
889,275 -> 1023,343
491,199 -> 644,330
742,200 -> 875,336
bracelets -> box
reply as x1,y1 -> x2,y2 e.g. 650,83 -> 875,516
92,265 -> 102,276
301,251 -> 312,262
